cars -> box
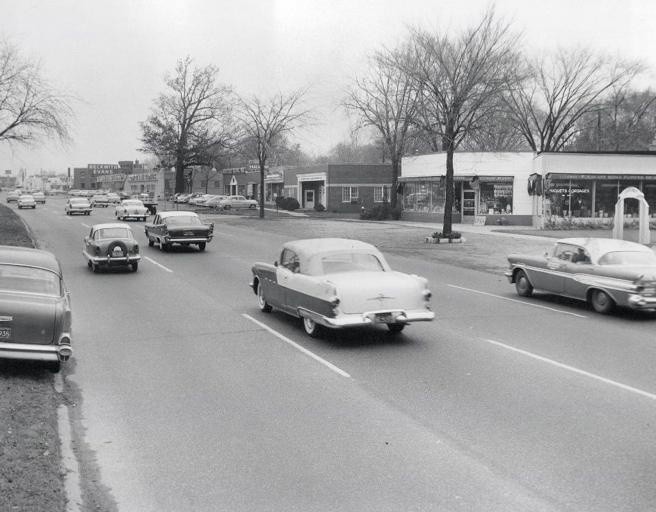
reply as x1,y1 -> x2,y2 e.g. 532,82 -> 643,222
144,210 -> 214,252
248,237 -> 435,339
504,237 -> 656,314
0,185 -> 259,222
0,245 -> 73,369
83,222 -> 140,272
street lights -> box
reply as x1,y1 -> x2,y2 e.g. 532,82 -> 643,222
171,165 -> 175,208
